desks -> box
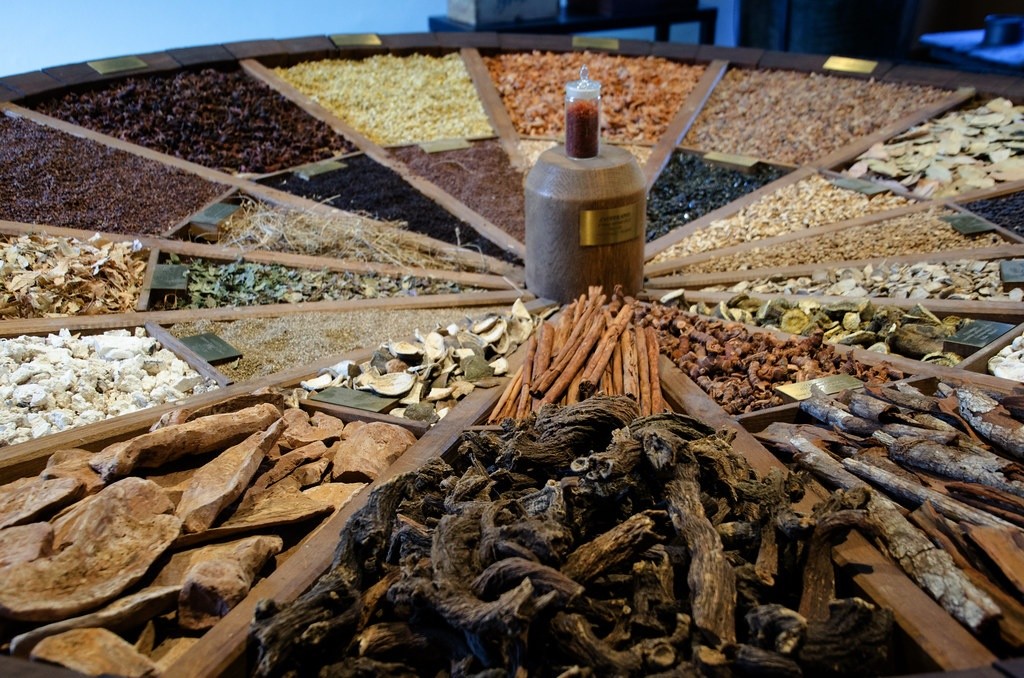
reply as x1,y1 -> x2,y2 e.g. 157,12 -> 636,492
428,6 -> 718,46
919,29 -> 1024,76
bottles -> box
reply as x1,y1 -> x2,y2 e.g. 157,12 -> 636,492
566,64 -> 601,162
